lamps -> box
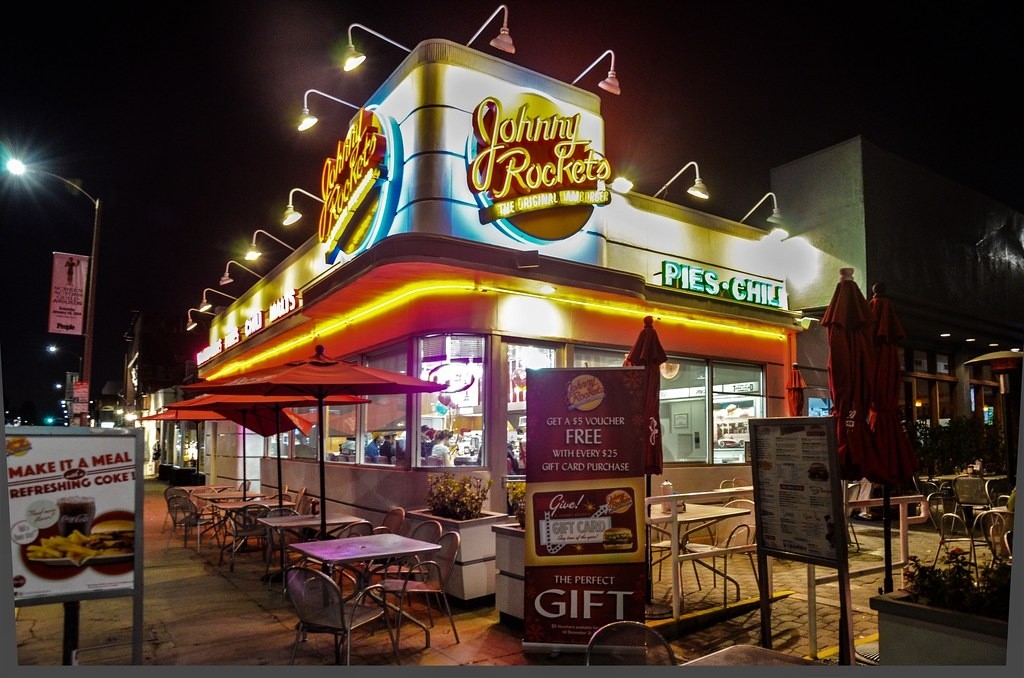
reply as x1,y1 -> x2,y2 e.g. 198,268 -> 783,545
653,161 -> 709,200
466,4 -> 515,54
571,50 -> 620,95
610,177 -> 633,194
343,23 -> 412,72
298,89 -> 360,132
245,230 -> 295,261
769,228 -> 789,241
514,251 -> 539,268
219,260 -> 262,285
186,308 -> 216,331
739,192 -> 784,223
199,288 -> 238,312
282,188 -> 325,225
795,317 -> 820,330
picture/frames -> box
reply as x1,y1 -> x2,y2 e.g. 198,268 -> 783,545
674,414 -> 688,428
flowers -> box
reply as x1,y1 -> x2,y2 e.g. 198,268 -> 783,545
899,548 -> 1012,626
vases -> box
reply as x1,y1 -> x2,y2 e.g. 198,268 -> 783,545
870,586 -> 1007,666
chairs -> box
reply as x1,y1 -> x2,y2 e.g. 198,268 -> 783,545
506,448 -> 526,476
584,621 -> 675,665
364,456 -> 396,465
162,480 -> 461,666
847,484 -> 861,549
652,477 -> 759,608
329,452 -> 337,461
913,475 -> 1015,589
421,457 -> 443,467
340,455 -> 355,463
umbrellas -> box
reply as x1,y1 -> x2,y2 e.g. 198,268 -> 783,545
134,344 -> 450,609
787,363 -> 808,417
328,403 -> 405,436
297,409 -> 342,423
623,317 -> 676,606
819,269 -> 920,593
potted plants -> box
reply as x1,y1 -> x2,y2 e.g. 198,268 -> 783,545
406,470 -> 508,610
491,482 -> 526,633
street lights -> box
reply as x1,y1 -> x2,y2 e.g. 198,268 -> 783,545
7,157 -> 101,387
48,345 -> 82,382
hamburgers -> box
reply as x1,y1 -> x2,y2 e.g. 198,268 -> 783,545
807,463 -> 828,482
88,520 -> 136,555
602,528 -> 634,550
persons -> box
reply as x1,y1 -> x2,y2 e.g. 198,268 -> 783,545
340,425 -> 524,475
1003,484 -> 1016,566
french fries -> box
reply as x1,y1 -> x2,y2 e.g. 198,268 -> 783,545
25,530 -> 98,564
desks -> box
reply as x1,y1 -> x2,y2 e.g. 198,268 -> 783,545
175,485 -> 235,493
193,492 -> 266,502
934,474 -> 1007,530
650,501 -> 751,614
257,513 -> 367,600
211,499 -> 296,510
676,644 -> 831,666
288,533 -> 442,665
714,484 -> 753,492
